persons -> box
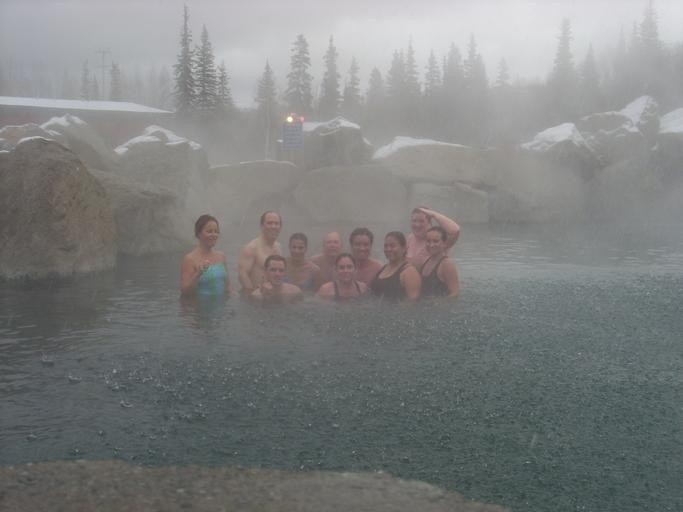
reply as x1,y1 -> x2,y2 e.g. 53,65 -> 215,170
251,255 -> 303,297
404,207 -> 461,275
238,211 -> 284,290
316,252 -> 368,297
349,228 -> 383,283
178,214 -> 234,295
283,232 -> 319,289
370,231 -> 420,301
417,226 -> 460,298
310,232 -> 345,281
